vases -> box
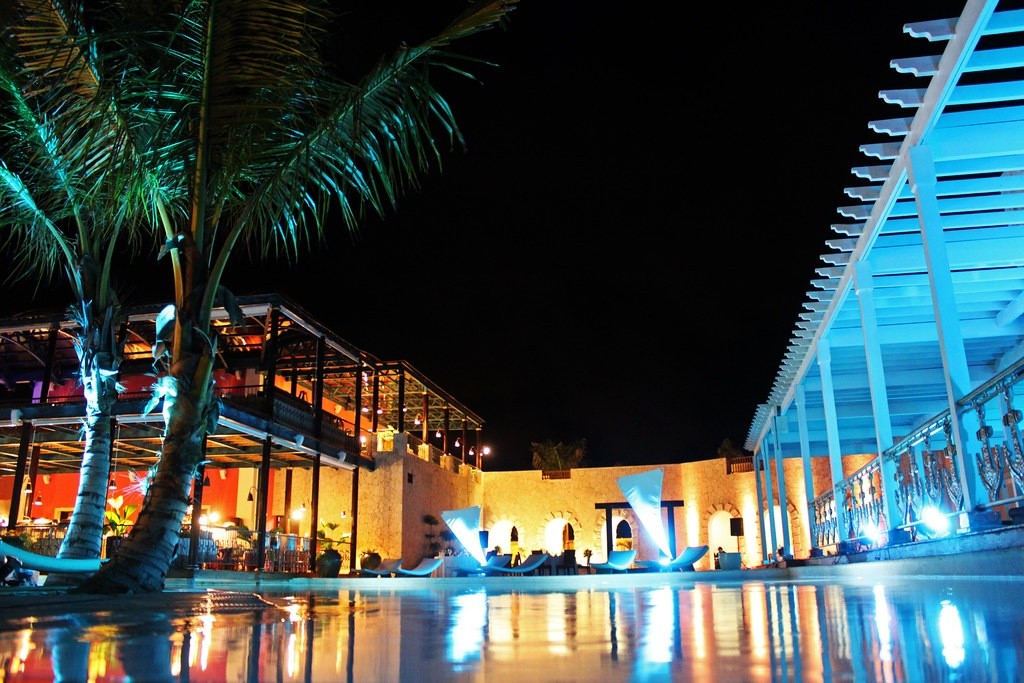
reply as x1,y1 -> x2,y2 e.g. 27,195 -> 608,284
718,553 -> 741,571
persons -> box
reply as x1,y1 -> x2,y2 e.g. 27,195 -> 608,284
47,519 -> 60,539
716,547 -> 726,569
431,547 -> 455,558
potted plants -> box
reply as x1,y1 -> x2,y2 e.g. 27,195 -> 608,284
358,549 -> 382,578
316,522 -> 349,578
422,515 -> 455,559
583,549 -> 592,574
104,495 -> 138,558
225,522 -> 284,572
1,530 -> 32,551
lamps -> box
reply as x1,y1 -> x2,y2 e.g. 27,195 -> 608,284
362,404 -> 369,412
107,473 -> 118,490
24,430 -> 36,493
436,428 -> 445,438
376,407 -> 382,415
414,414 -> 423,424
305,374 -> 314,381
301,498 -> 310,511
454,438 -> 463,447
341,507 -> 351,519
246,486 -> 257,501
203,470 -> 211,486
35,496 -> 42,505
469,446 -> 477,455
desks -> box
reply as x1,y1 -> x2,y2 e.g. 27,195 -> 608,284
106,536 -> 218,570
532,557 -> 577,575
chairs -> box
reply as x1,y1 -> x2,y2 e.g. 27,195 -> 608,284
634,545 -> 710,573
351,558 -> 403,578
588,550 -> 638,574
447,555 -> 511,578
504,550 -> 576,576
385,557 -> 443,577
480,553 -> 549,577
